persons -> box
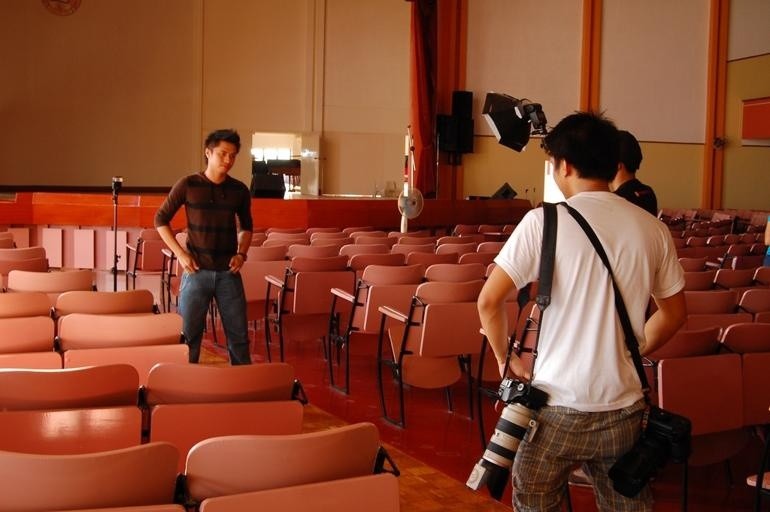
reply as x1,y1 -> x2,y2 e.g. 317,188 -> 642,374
476,110 -> 688,512
608,130 -> 658,218
762,215 -> 770,267
155,129 -> 252,366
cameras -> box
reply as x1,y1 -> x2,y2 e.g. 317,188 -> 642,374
466,377 -> 551,502
608,406 -> 692,498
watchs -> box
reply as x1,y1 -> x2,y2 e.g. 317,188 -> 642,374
237,253 -> 248,261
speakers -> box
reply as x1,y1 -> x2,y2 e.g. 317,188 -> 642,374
433,90 -> 473,157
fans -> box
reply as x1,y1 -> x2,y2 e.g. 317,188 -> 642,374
397,187 -> 425,233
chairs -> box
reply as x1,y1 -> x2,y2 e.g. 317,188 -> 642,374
376,278 -> 487,431
677,254 -> 709,273
349,253 -> 406,289
54,289 -> 160,325
648,289 -> 753,334
718,243 -> 752,271
355,235 -> 398,250
249,238 -> 264,248
1,245 -> 47,261
391,243 -> 435,264
745,243 -> 763,253
251,226 -> 263,233
257,262 -> 318,274
0,315 -> 64,370
642,284 -> 742,512
502,224 -> 518,240
305,227 -> 339,239
0,237 -> 17,249
252,232 -> 265,240
453,224 -> 480,237
0,363 -> 142,456
707,234 -> 726,246
398,236 -> 438,246
687,234 -> 707,247
670,235 -> 687,248
712,266 -> 753,291
471,280 -> 541,451
1,441 -> 181,512
387,230 -> 432,244
246,244 -> 288,262
6,268 -> 97,307
343,226 -> 375,237
436,241 -> 478,263
123,226 -> 167,290
425,262 -> 485,283
0,230 -> 15,239
137,360 -> 308,474
477,224 -> 500,241
172,420 -> 400,512
739,287 -> 770,323
160,231 -> 187,314
721,322 -> 770,511
679,269 -> 717,292
406,252 -> 459,277
459,251 -> 500,268
749,242 -> 761,255
263,253 -> 355,364
726,234 -> 740,245
328,263 -> 427,396
0,290 -> 53,319
338,243 -> 390,267
740,232 -> 756,244
288,243 -> 340,260
239,260 -> 291,323
265,227 -> 307,237
349,232 -> 387,242
436,234 -> 485,247
753,265 -> 768,287
0,257 -> 50,291
311,237 -> 354,246
476,241 -> 506,253
267,231 -> 311,241
310,232 -> 347,243
484,261 -> 497,277
263,237 -> 310,247
54,312 -> 188,388
